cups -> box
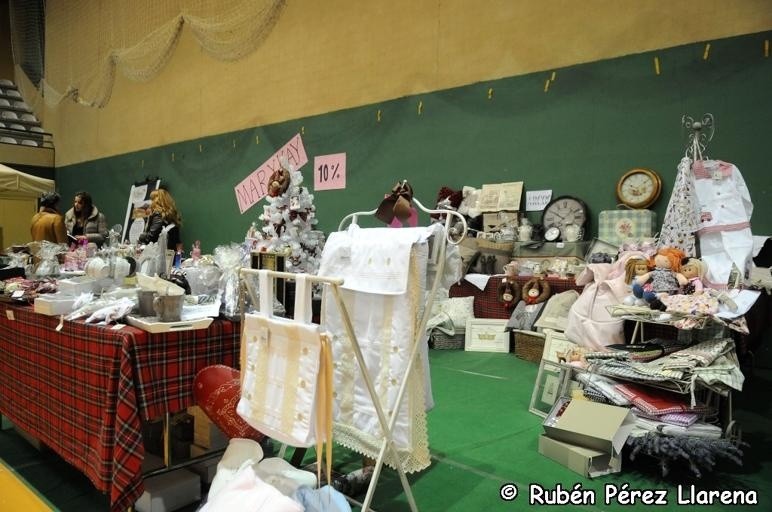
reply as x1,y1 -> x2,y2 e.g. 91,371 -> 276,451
135,288 -> 159,317
566,227 -> 582,243
114,256 -> 131,280
84,256 -> 110,281
151,288 -> 184,323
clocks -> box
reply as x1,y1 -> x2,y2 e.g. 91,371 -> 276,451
541,195 -> 589,241
617,169 -> 663,209
544,227 -> 561,241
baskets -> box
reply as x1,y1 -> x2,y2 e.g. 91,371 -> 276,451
478,239 -> 513,252
433,333 -> 464,350
515,333 -> 544,362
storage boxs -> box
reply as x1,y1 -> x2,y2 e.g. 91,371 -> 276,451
598,209 -> 657,249
483,212 -> 518,233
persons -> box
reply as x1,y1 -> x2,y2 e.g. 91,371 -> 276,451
620,257 -> 653,307
136,188 -> 184,265
28,190 -> 70,272
63,190 -> 110,250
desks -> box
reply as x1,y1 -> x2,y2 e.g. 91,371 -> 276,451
0,296 -> 239,512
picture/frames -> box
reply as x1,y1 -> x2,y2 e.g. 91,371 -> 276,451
542,331 -> 577,372
528,358 -> 571,418
465,319 -> 511,354
